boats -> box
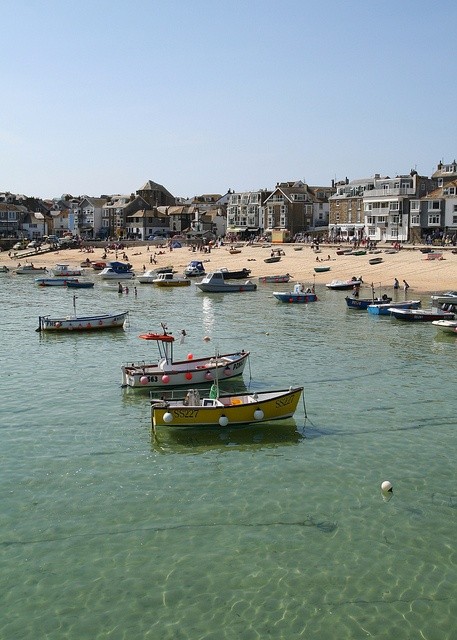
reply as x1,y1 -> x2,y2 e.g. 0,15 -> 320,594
345,296 -> 392,310
314,266 -> 330,272
81,258 -> 95,267
432,320 -> 457,332
0,266 -> 9,273
431,291 -> 457,312
39,311 -> 129,331
66,281 -> 95,288
194,272 -> 257,291
14,263 -> 48,274
387,306 -> 456,322
337,249 -> 352,254
184,260 -> 205,276
264,257 -> 280,263
230,250 -> 242,253
35,278 -> 78,287
137,267 -> 173,284
51,264 -> 81,276
369,258 -> 383,264
259,274 -> 290,283
98,262 -> 135,280
294,247 -> 303,251
221,267 -> 250,280
150,387 -> 305,427
367,300 -> 421,315
121,323 -> 250,388
153,272 -> 191,287
353,250 -> 367,256
272,291 -> 316,302
325,279 -> 361,290
91,262 -> 110,270
423,253 -> 444,259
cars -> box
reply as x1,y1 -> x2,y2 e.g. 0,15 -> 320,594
13,242 -> 27,250
28,241 -> 41,248
47,235 -> 59,243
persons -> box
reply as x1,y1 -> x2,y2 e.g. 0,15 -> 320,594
124,287 -> 128,294
1,225 -> 456,274
402,280 -> 409,292
133,286 -> 137,295
393,278 -> 399,289
350,275 -> 364,284
352,284 -> 359,299
117,281 -> 122,293
